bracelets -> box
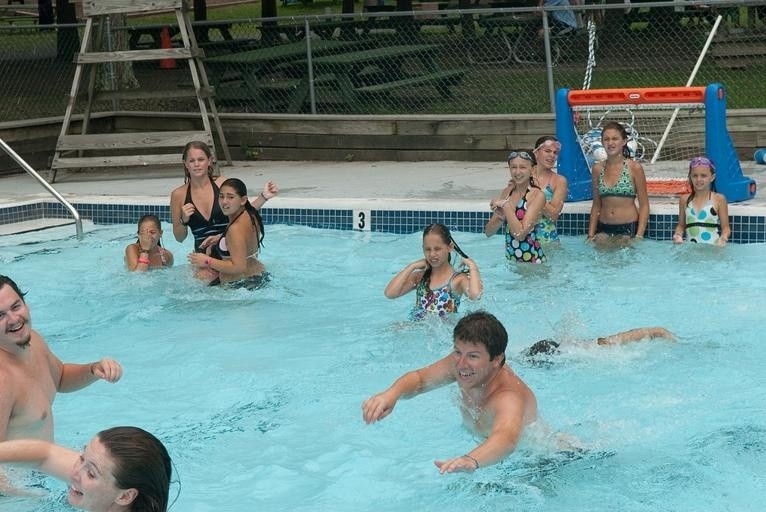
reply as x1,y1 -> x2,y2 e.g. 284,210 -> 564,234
138,258 -> 151,264
501,200 -> 509,208
463,455 -> 478,470
138,249 -> 152,254
204,256 -> 214,267
91,361 -> 101,375
260,193 -> 269,203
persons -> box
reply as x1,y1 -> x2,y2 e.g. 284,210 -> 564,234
170,140 -> 278,266
476,0 -> 766,68
511,135 -> 568,246
672,156 -> 732,248
584,123 -> 650,241
0,426 -> 181,512
485,149 -> 547,267
384,223 -> 483,324
0,275 -> 123,495
126,215 -> 174,273
528,326 -> 694,365
362,310 -> 593,475
188,177 -> 270,294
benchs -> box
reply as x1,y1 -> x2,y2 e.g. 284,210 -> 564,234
259,66 -> 386,113
351,69 -> 468,105
539,25 -> 601,41
176,63 -> 289,107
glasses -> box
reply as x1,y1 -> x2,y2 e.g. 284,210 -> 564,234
689,157 -> 716,170
532,139 -> 561,154
508,152 -> 537,165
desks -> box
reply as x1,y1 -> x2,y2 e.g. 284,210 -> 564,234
285,44 -> 457,113
256,20 -> 365,40
127,24 -> 233,43
202,39 -> 373,113
467,15 -> 560,66
